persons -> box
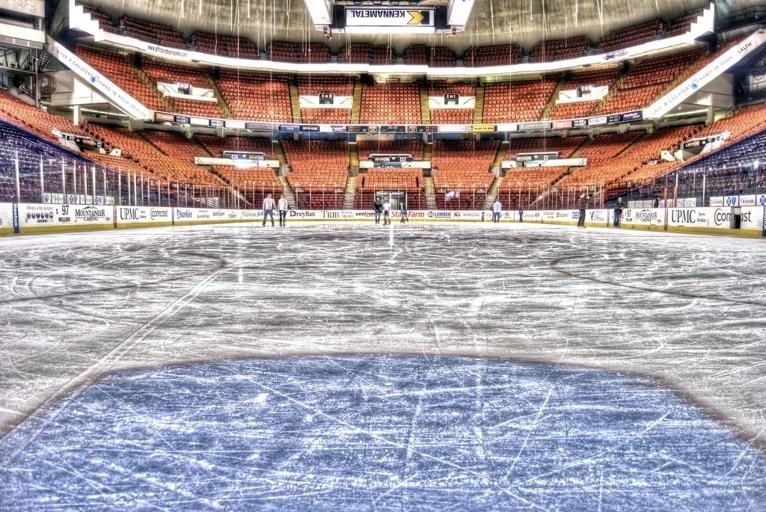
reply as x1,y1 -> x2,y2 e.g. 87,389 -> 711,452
399,201 -> 409,223
276,194 -> 288,228
492,199 -> 502,223
577,192 -> 590,228
383,199 -> 392,224
262,193 -> 278,227
613,196 -> 624,228
373,197 -> 382,224
519,203 -> 524,222
654,198 -> 659,208
